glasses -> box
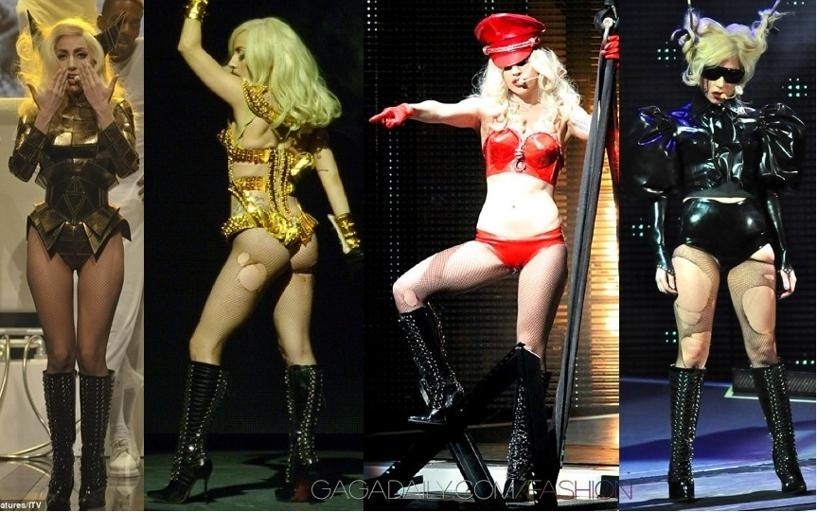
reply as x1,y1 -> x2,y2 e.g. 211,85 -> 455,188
703,63 -> 742,89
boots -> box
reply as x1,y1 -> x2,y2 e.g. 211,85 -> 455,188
503,343 -> 552,502
396,300 -> 465,426
748,359 -> 806,494
147,362 -> 230,505
43,371 -> 77,508
666,365 -> 706,502
80,370 -> 114,511
274,362 -> 326,504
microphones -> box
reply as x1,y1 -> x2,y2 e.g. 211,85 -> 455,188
720,93 -> 738,101
517,75 -> 539,85
74,75 -> 80,82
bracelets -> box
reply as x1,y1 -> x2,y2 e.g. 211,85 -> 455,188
184,0 -> 208,22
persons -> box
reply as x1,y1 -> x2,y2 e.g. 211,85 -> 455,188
9,0 -> 144,511
369,12 -> 620,502
627,8 -> 807,506
146,0 -> 364,506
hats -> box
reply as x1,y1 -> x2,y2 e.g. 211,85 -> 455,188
475,12 -> 546,70
